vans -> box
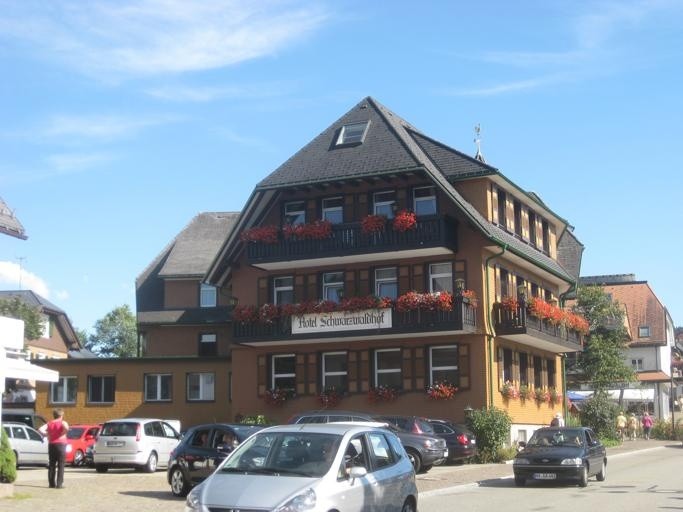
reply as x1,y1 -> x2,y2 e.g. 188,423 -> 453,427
0,408 -> 49,432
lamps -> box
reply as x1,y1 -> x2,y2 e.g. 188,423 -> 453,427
517,282 -> 528,306
453,277 -> 466,298
229,295 -> 240,313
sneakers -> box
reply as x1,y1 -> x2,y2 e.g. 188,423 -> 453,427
49,482 -> 63,489
617,437 -> 651,442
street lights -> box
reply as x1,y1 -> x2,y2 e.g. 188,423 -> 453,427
670,365 -> 680,429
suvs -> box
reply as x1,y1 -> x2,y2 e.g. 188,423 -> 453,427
284,409 -> 449,479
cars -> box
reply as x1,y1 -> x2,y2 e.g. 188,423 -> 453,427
165,422 -> 297,499
63,421 -> 106,469
424,415 -> 478,468
512,426 -> 608,488
372,413 -> 436,436
84,444 -> 96,468
3,419 -> 57,470
91,417 -> 188,473
180,418 -> 421,511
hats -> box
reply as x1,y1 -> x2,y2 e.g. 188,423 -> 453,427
556,413 -> 561,418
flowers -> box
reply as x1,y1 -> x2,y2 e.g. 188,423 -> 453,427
231,286 -> 480,326
260,378 -> 461,409
494,289 -> 591,340
499,379 -> 573,411
238,204 -> 418,246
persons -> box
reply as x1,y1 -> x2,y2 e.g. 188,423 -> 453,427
36,409 -> 70,489
616,411 -> 653,440
551,414 -> 564,425
